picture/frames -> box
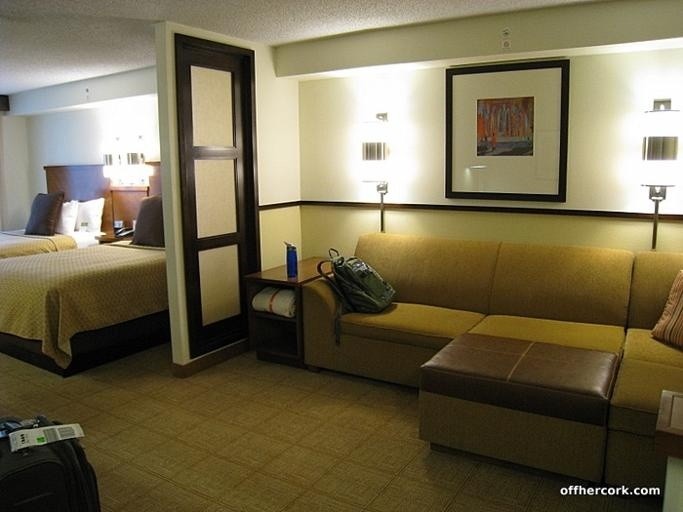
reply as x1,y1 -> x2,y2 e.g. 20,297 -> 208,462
444,59 -> 569,201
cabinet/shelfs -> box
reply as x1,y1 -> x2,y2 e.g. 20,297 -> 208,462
244,257 -> 328,367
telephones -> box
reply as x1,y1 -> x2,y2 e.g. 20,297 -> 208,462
114,227 -> 132,238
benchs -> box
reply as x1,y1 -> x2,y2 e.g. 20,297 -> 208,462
419,333 -> 620,487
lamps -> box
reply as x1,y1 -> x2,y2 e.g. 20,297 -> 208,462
360,132 -> 394,232
639,111 -> 678,249
103,153 -> 121,183
127,152 -> 145,179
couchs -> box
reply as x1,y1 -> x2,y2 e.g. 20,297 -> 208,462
302,232 -> 683,491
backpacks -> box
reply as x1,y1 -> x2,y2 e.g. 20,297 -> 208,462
319,248 -> 397,314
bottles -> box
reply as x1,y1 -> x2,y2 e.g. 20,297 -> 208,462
286,244 -> 298,279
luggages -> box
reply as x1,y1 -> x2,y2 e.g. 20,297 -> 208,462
0,414 -> 103,511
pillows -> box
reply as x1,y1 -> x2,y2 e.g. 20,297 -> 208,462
130,194 -> 164,247
651,270 -> 683,347
24,192 -> 105,236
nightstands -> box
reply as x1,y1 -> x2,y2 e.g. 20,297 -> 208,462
95,232 -> 133,244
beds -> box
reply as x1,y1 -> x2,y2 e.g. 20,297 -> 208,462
0,240 -> 168,378
0,229 -> 106,258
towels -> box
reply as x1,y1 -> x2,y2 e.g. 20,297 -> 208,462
252,286 -> 296,318
